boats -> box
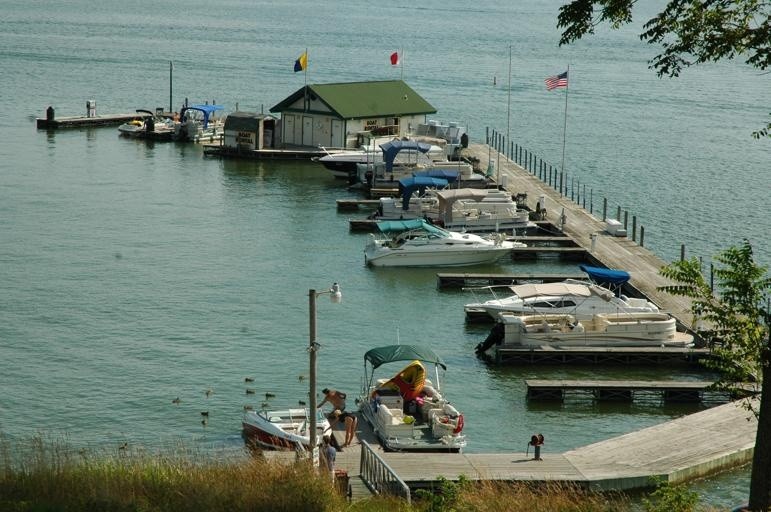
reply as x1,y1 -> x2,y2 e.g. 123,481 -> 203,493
312,137 -> 535,229
118,102 -> 224,141
242,407 -> 332,451
464,278 -> 694,346
363,219 -> 526,266
358,343 -> 466,452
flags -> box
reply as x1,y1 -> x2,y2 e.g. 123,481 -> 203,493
294,52 -> 307,73
390,48 -> 405,66
545,71 -> 568,91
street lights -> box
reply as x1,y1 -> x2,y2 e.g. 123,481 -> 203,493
306,282 -> 342,448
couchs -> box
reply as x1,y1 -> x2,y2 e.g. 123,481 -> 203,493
428,404 -> 460,439
379,405 -> 414,438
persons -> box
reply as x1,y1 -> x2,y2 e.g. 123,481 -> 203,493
325,409 -> 360,448
319,434 -> 338,489
317,387 -> 347,414
